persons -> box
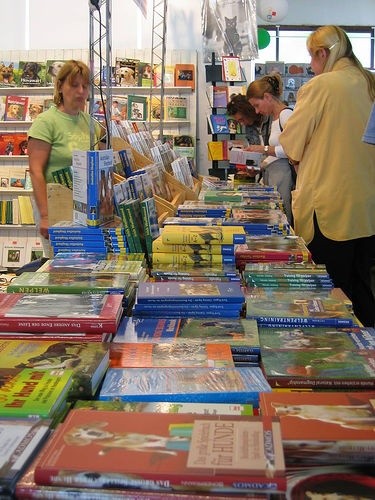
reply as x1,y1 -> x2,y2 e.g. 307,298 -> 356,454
26,61 -> 106,259
243,74 -> 293,226
227,94 -> 297,230
278,25 -> 375,330
362,99 -> 375,146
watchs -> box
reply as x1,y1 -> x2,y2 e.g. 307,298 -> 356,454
264,146 -> 269,157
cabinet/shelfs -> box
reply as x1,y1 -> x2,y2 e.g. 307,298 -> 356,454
205,51 -> 246,180
0,48 -> 203,272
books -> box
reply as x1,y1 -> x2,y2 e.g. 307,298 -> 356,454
0,57 -> 375,500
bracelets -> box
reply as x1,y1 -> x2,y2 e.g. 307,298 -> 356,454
40,214 -> 48,220
